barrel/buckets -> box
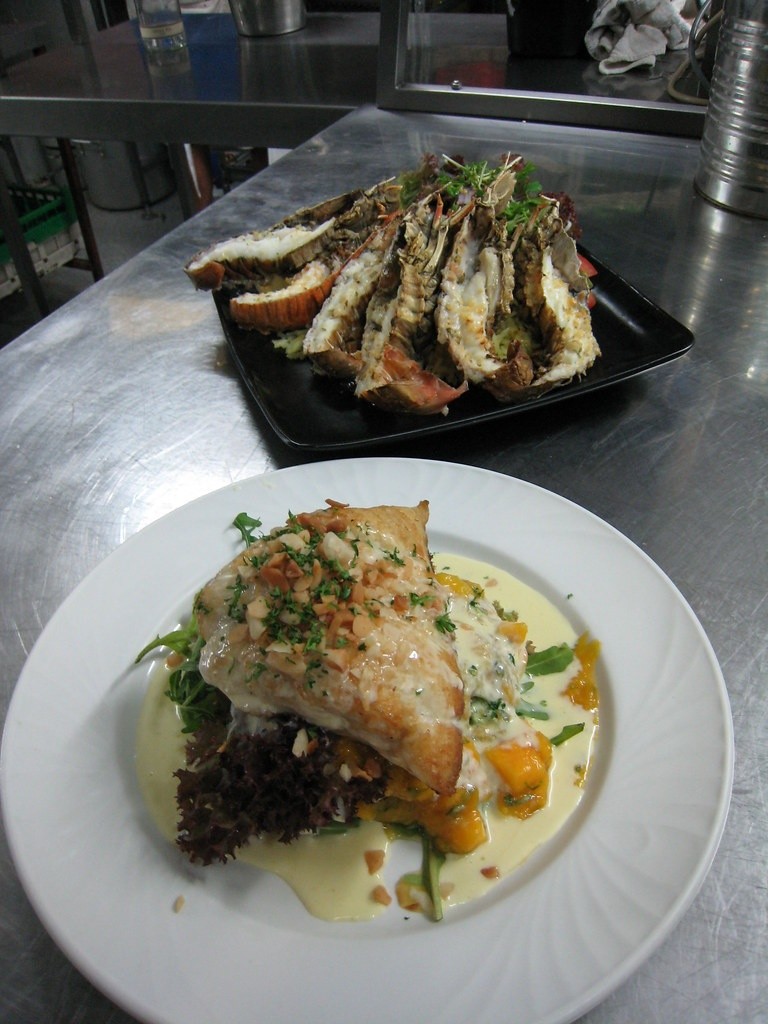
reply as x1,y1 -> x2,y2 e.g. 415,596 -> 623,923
72,139 -> 174,211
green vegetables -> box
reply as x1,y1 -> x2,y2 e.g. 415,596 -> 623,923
392,154 -> 556,236
132,514 -> 589,923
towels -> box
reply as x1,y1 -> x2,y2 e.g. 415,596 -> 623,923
584,0 -> 711,75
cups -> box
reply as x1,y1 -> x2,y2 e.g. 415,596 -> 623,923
507,0 -> 598,56
693,11 -> 768,220
229,0 -> 306,37
134,0 -> 186,51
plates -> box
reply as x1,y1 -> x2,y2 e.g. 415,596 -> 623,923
0,457 -> 733,1024
212,244 -> 695,455
